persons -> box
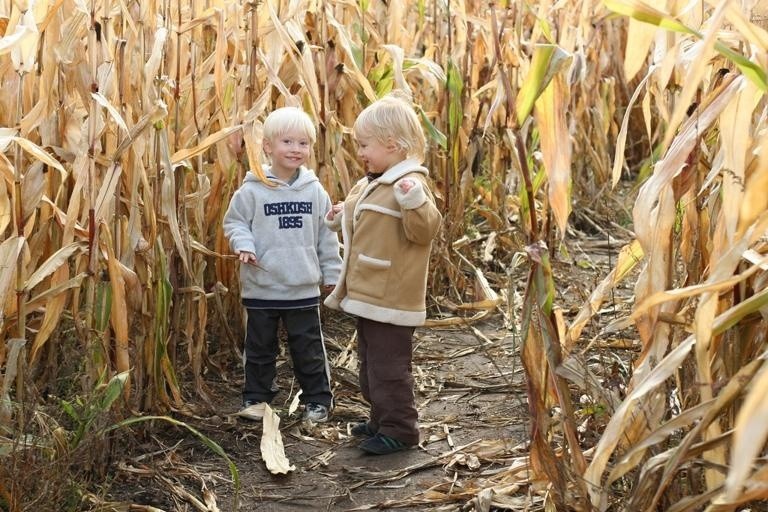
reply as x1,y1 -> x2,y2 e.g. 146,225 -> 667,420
321,94 -> 441,455
222,107 -> 343,424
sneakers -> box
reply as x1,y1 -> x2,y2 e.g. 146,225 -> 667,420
302,403 -> 328,423
352,424 -> 408,454
